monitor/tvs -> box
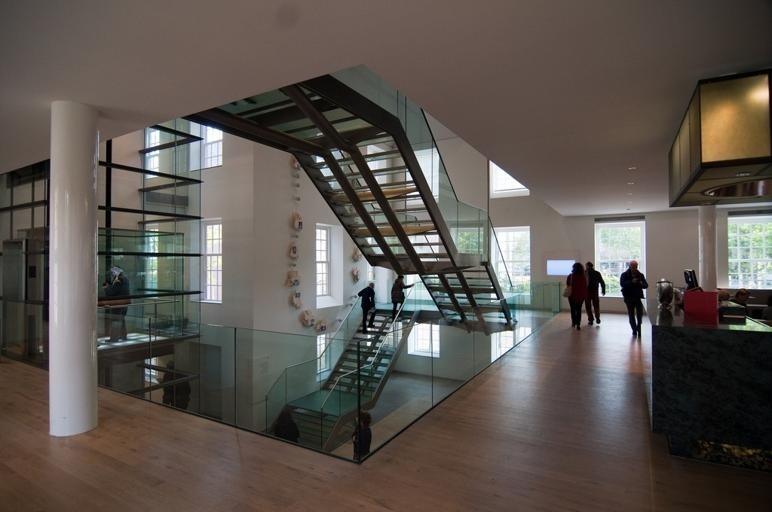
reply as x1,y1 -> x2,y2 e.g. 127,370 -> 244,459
683,269 -> 698,290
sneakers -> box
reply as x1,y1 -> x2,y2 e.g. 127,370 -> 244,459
570,318 -> 643,339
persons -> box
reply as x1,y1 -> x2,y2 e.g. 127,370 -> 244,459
718,289 -> 730,304
582,261 -> 605,326
357,282 -> 377,333
157,359 -> 193,411
102,266 -> 130,343
352,412 -> 372,460
729,288 -> 751,317
762,294 -> 772,321
565,262 -> 589,330
619,260 -> 649,339
270,409 -> 301,444
391,274 -> 415,322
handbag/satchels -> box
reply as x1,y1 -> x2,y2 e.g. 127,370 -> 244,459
561,285 -> 573,298
